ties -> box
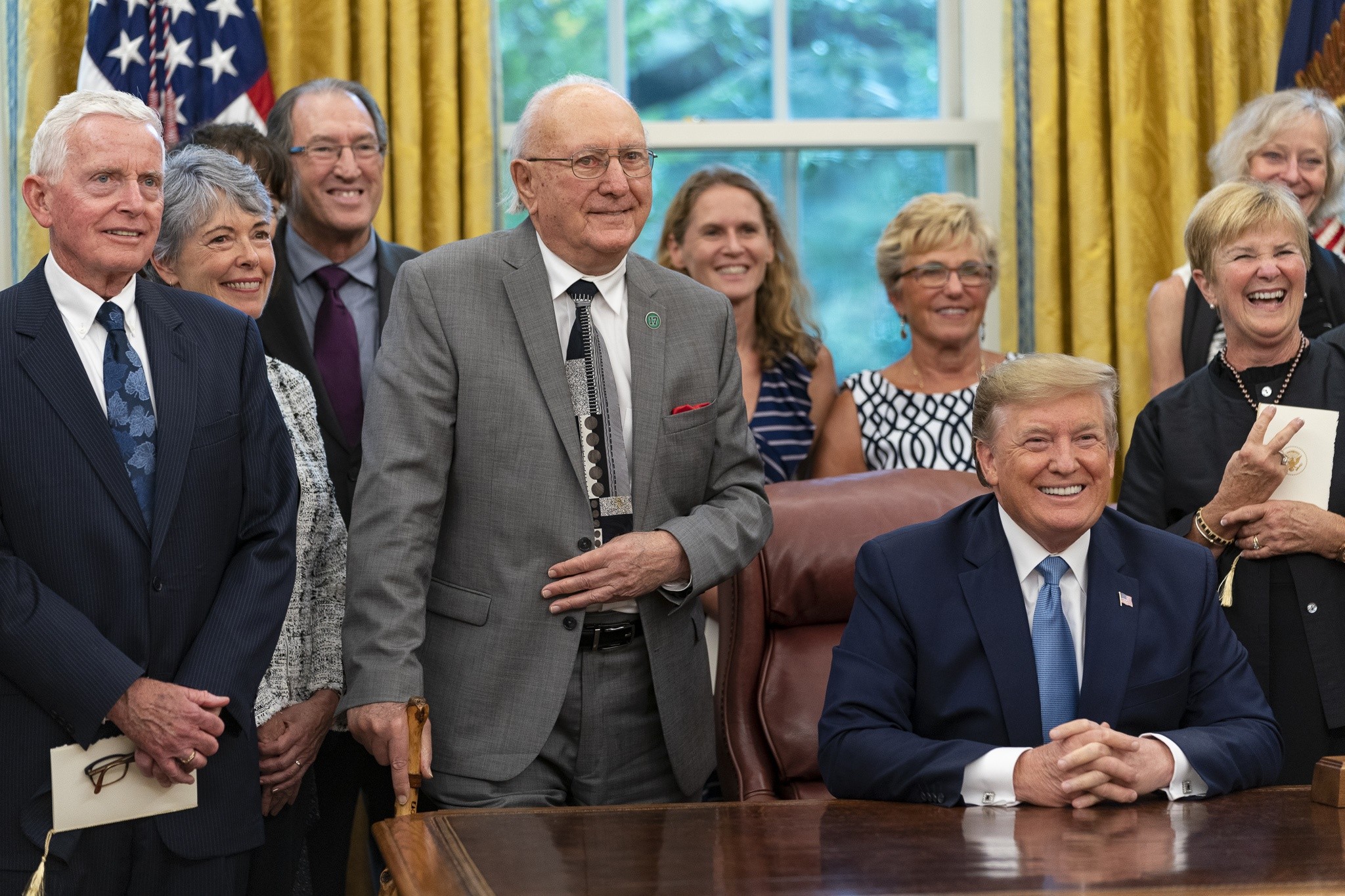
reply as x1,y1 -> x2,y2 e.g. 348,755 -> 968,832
562,279 -> 634,548
312,264 -> 365,454
1032,556 -> 1081,746
96,302 -> 158,535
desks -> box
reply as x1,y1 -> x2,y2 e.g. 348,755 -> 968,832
374,784 -> 1345,895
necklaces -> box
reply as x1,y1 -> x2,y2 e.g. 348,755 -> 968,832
1220,330 -> 1305,411
909,346 -> 986,394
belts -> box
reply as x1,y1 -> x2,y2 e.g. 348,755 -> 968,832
578,621 -> 643,652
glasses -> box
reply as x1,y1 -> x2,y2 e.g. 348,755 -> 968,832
288,143 -> 387,165
84,749 -> 136,794
526,147 -> 658,179
896,264 -> 994,289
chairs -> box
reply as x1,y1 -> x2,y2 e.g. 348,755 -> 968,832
715,467 -> 993,802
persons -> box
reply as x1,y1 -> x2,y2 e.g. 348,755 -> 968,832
817,353 -> 1283,812
817,192 -> 1029,479
330,74 -> 773,809
1147,89 -> 1345,395
142,78 -> 420,896
656,163 -> 836,696
0,90 -> 302,895
1117,177 -> 1345,786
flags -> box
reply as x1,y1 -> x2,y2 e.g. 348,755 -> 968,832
78,0 -> 275,145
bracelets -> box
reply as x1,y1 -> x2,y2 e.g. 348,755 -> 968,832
1195,507 -> 1235,547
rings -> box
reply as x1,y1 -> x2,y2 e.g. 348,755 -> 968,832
1278,451 -> 1289,465
295,760 -> 302,768
1253,535 -> 1262,550
177,750 -> 195,764
